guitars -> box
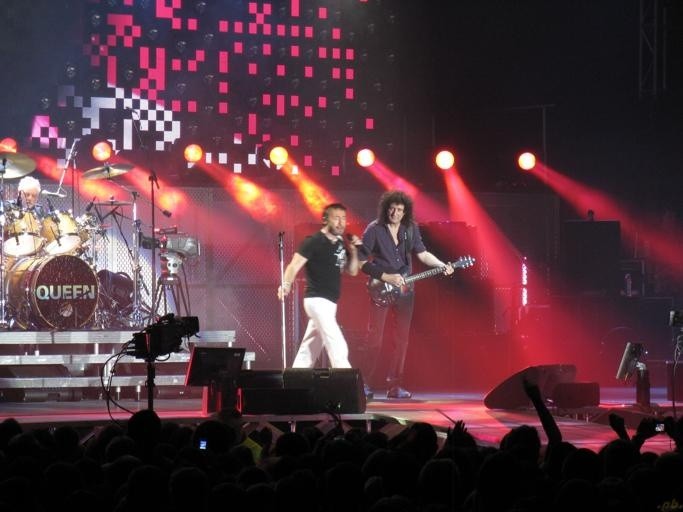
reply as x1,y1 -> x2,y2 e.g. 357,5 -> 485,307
366,255 -> 476,307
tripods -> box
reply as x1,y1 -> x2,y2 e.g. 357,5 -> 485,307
93,107 -> 172,331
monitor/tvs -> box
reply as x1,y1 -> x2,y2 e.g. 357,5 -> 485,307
184,346 -> 245,387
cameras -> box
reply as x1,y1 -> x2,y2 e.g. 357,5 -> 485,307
199,440 -> 208,451
654,422 -> 666,433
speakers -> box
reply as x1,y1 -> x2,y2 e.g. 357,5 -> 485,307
283,366 -> 366,414
223,370 -> 284,415
484,363 -> 576,409
552,382 -> 601,408
561,218 -> 620,296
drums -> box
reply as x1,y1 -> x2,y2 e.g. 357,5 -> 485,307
3,254 -> 99,329
40,210 -> 82,253
3,208 -> 45,257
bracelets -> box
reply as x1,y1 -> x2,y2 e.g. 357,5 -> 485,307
282,282 -> 291,289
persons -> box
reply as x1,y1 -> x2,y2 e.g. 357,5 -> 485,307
0,176 -> 46,237
275,203 -> 363,369
340,189 -> 455,401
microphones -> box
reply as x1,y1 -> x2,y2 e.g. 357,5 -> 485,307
16,191 -> 22,207
346,232 -> 372,257
85,196 -> 96,211
42,190 -> 67,198
48,201 -> 57,221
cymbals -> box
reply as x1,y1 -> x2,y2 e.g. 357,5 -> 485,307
0,151 -> 36,179
93,200 -> 132,205
82,164 -> 134,180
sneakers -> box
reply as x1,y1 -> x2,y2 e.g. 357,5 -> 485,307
364,384 -> 373,398
386,386 -> 412,398
